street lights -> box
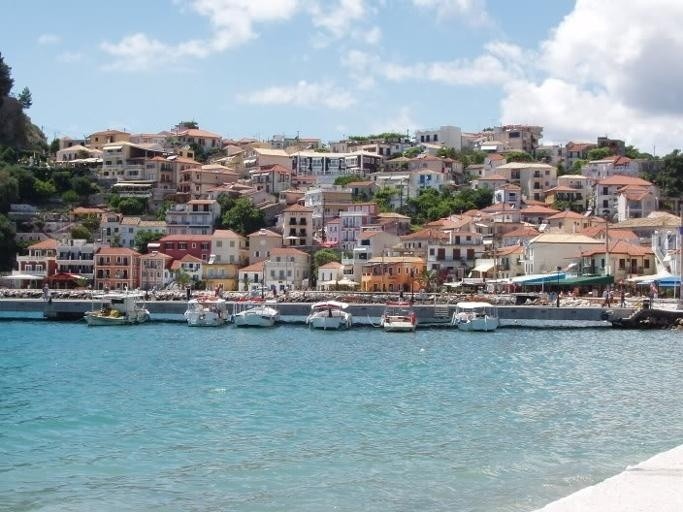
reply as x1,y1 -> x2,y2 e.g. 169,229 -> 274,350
410,266 -> 416,302
555,265 -> 561,306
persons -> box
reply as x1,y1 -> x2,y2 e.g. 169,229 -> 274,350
619,292 -> 625,308
599,290 -> 610,308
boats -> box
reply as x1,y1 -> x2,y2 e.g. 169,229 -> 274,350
231,268 -> 277,329
84,291 -> 155,326
182,294 -> 231,327
451,301 -> 500,331
382,301 -> 419,331
306,299 -> 355,330
